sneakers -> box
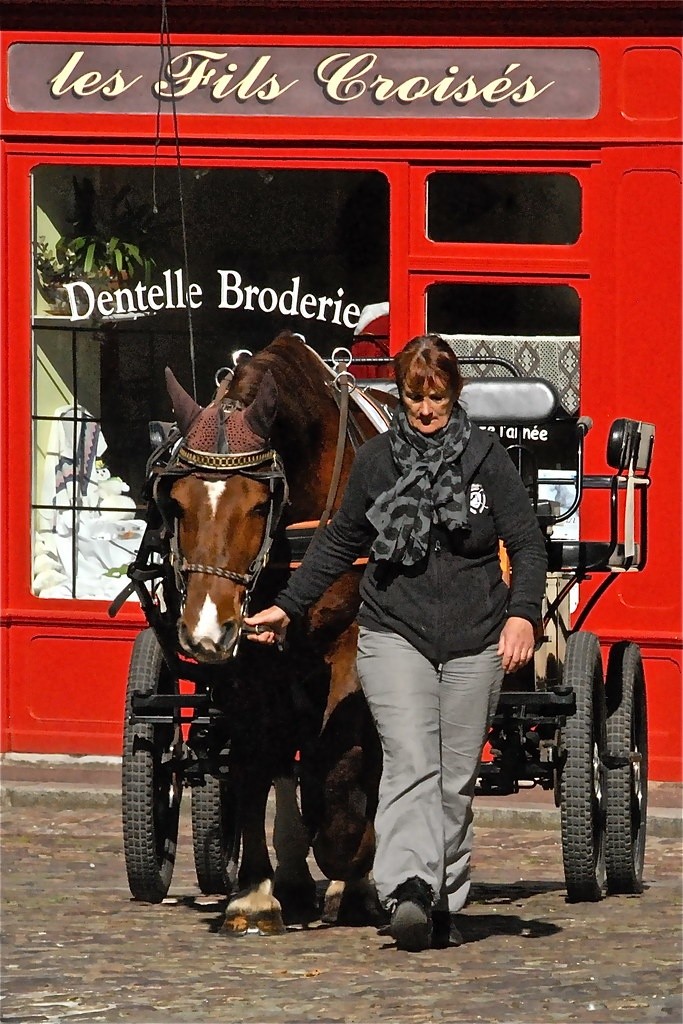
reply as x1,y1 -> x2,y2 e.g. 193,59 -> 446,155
386,878 -> 434,945
437,913 -> 464,946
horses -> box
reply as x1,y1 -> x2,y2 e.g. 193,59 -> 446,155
159,329 -> 402,935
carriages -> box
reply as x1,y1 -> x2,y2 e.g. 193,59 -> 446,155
108,328 -> 656,936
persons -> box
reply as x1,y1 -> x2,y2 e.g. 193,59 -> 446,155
244,335 -> 547,948
